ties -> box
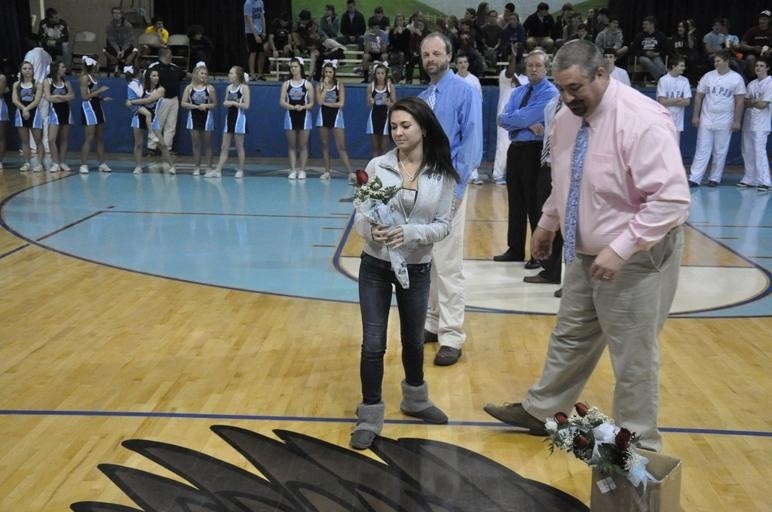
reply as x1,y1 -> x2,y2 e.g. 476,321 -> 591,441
425,86 -> 437,111
541,95 -> 561,168
510,85 -> 532,139
564,122 -> 589,266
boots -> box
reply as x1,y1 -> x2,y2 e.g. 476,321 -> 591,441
350,402 -> 385,449
399,379 -> 448,424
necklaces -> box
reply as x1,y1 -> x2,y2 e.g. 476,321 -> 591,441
400,161 -> 418,182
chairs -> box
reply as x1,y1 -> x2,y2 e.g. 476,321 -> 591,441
627,38 -> 772,88
70,30 -> 190,80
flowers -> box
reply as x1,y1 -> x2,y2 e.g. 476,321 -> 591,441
339,170 -> 411,290
543,403 -> 657,488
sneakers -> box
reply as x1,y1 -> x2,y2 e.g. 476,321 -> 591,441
99,163 -> 111,172
434,345 -> 462,366
148,131 -> 159,142
424,329 -> 438,344
347,172 -> 357,181
169,166 -> 176,174
19,161 -> 69,173
688,181 -> 772,191
288,169 -> 308,179
80,165 -> 89,174
133,167 -> 143,173
471,177 -> 506,184
319,172 -> 330,180
189,164 -> 244,179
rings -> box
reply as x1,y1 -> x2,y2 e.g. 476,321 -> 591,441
604,275 -> 610,281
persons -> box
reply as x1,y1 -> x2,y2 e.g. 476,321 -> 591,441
243,1 -> 772,94
416,32 -> 484,366
350,95 -> 462,448
492,48 -> 560,268
205,66 -> 251,177
657,55 -> 694,131
315,60 -> 357,179
483,37 -> 690,451
366,60 -> 397,157
181,62 -> 219,177
0,6 -> 193,176
687,51 -> 747,186
455,56 -> 484,184
280,57 -> 315,179
736,59 -> 771,191
492,41 -> 531,184
524,93 -> 566,297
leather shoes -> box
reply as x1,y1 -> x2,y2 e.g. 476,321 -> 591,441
493,254 -> 562,297
484,402 -> 551,436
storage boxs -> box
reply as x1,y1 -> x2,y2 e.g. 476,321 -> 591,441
590,447 -> 682,511
